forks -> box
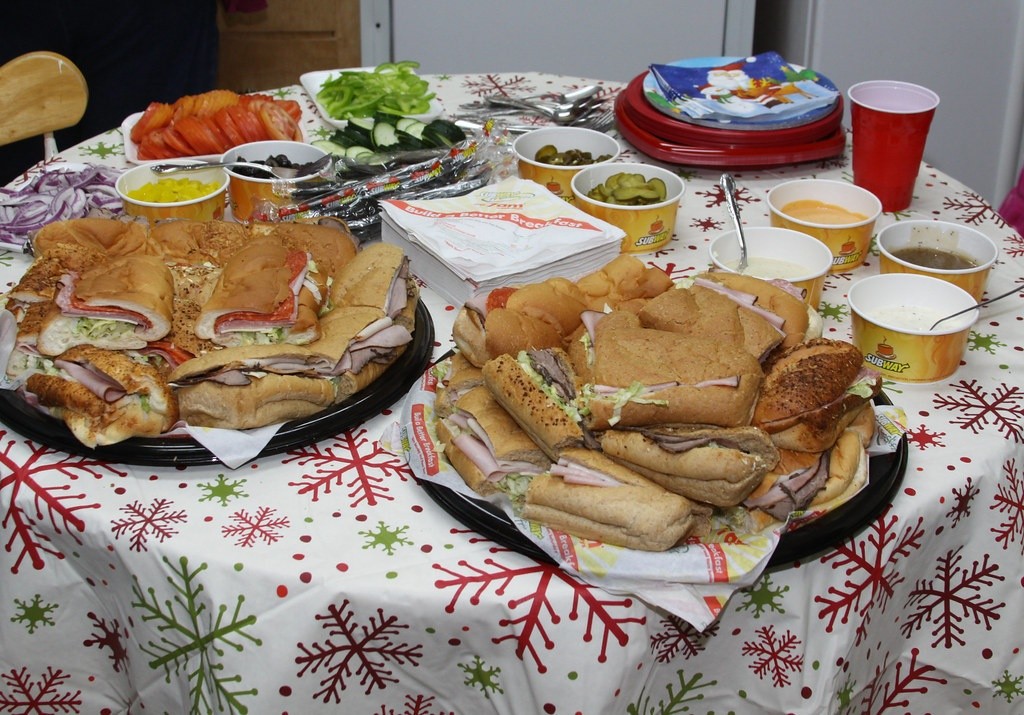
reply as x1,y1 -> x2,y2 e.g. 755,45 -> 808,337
455,107 -> 618,140
151,152 -> 332,178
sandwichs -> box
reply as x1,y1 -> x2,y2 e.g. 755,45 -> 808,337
437,256 -> 877,551
5,219 -> 409,445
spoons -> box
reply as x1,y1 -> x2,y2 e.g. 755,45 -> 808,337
486,85 -> 603,125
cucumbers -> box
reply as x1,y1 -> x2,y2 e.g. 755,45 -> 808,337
311,112 -> 464,162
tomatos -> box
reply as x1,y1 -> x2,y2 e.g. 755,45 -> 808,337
131,89 -> 303,159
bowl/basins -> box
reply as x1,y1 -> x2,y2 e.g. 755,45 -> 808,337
571,163 -> 685,253
220,140 -> 332,223
767,179 -> 882,273
115,159 -> 230,229
512,127 -> 620,209
848,273 -> 980,383
876,220 -> 998,305
709,227 -> 832,311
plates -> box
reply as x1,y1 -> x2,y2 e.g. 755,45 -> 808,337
122,112 -> 309,166
612,57 -> 845,169
415,346 -> 907,569
0,298 -> 434,467
299,67 -> 439,130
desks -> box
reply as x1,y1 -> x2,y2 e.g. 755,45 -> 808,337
0,72 -> 1024,715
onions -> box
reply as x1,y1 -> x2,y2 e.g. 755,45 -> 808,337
0,165 -> 124,246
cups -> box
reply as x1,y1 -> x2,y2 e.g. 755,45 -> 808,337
848,80 -> 939,212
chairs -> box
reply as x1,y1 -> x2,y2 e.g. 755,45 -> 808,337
1,51 -> 89,161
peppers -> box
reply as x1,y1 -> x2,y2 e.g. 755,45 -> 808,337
319,61 -> 433,118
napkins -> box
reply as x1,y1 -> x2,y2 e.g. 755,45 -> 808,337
378,176 -> 627,310
